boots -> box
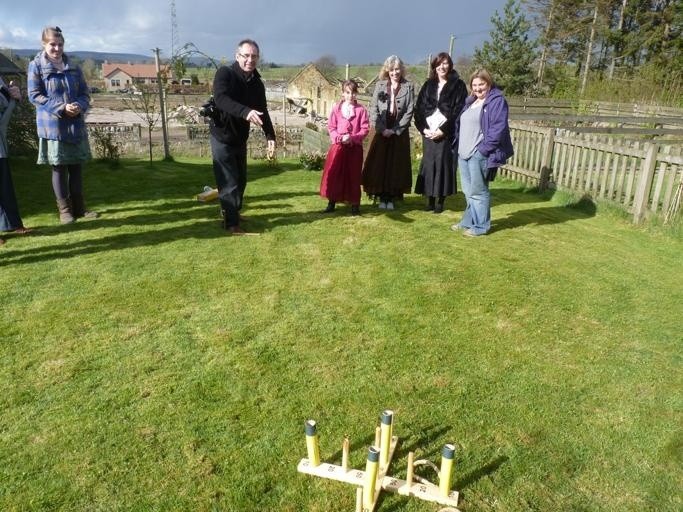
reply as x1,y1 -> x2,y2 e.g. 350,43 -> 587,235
69,193 -> 97,218
419,197 -> 447,214
56,197 -> 73,221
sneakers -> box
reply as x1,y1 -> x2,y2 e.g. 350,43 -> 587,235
351,204 -> 362,216
376,201 -> 394,210
221,212 -> 248,236
318,200 -> 336,214
1,225 -> 34,235
450,223 -> 482,237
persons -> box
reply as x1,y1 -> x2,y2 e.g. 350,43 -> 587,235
361,55 -> 414,211
318,79 -> 370,217
413,52 -> 468,214
0,74 -> 35,245
209,38 -> 277,236
451,69 -> 514,238
27,26 -> 99,224
